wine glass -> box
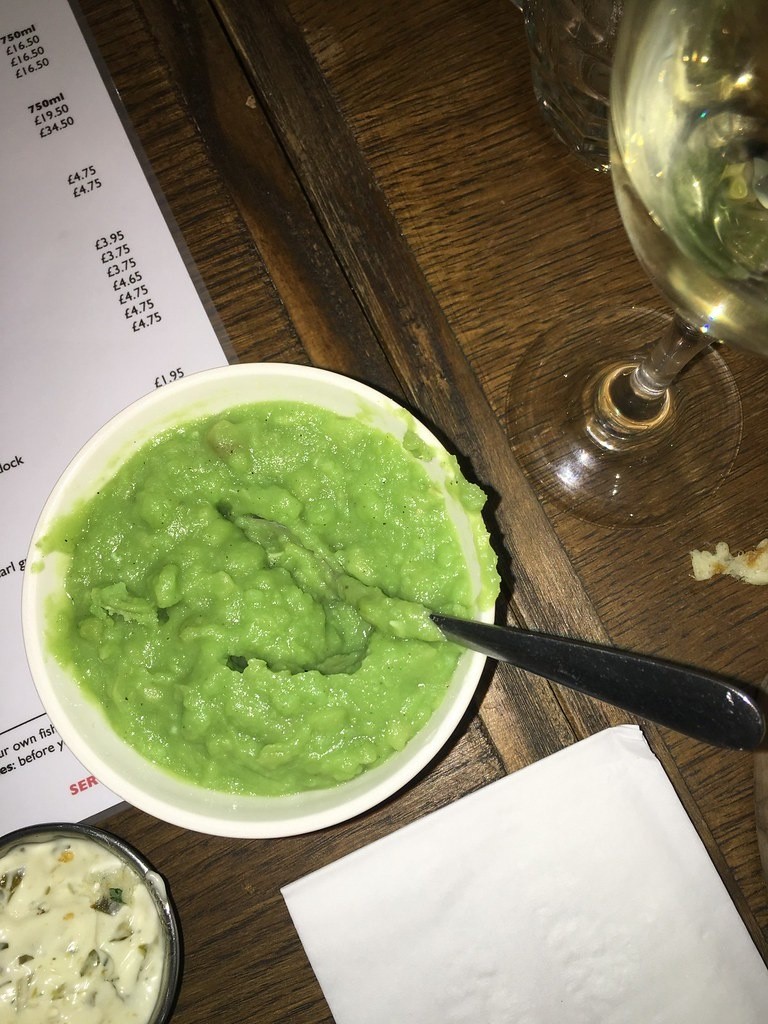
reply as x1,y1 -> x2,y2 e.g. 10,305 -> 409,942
505,0 -> 768,531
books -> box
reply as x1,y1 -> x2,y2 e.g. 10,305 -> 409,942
282,725 -> 768,1024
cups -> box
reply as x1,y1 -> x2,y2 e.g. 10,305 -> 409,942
523,0 -> 644,172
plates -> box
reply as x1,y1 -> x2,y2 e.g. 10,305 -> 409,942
18,363 -> 498,839
0,820 -> 183,1024
